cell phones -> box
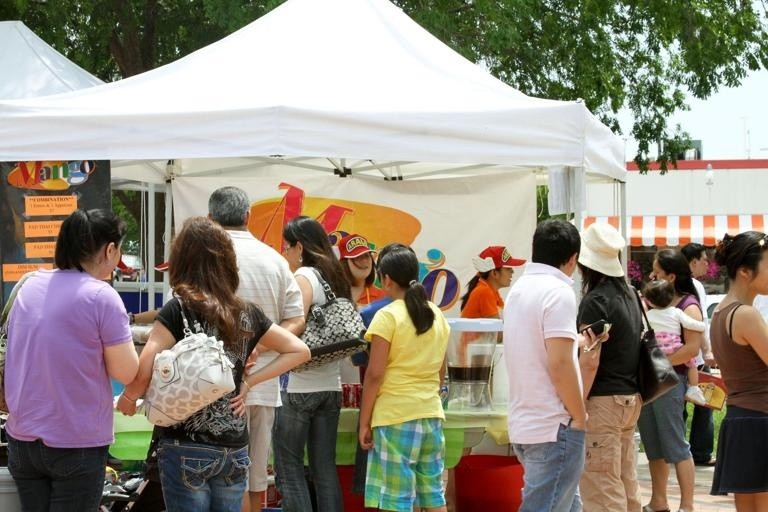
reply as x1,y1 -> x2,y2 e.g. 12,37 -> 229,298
581,319 -> 607,337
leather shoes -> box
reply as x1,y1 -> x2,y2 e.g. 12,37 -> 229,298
696,459 -> 716,467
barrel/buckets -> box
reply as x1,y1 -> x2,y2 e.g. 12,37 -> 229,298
455,454 -> 524,512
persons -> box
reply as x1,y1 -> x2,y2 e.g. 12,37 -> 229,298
202,185 -> 309,511
453,244 -> 528,368
1,206 -> 140,511
495,218 -> 715,512
710,232 -> 768,511
355,239 -> 451,512
271,212 -> 355,511
347,241 -> 417,496
340,233 -> 389,310
127,260 -> 170,326
112,214 -> 313,511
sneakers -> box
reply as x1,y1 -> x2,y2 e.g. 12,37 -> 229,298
685,383 -> 706,407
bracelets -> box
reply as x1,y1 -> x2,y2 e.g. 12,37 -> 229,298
128,311 -> 135,326
241,378 -> 253,392
119,391 -> 140,404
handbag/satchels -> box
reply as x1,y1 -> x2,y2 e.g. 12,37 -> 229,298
685,370 -> 727,410
144,333 -> 236,428
291,297 -> 368,373
0,334 -> 9,415
638,329 -> 680,406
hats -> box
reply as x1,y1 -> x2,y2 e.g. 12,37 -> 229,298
472,246 -> 527,273
578,222 -> 625,277
332,234 -> 376,260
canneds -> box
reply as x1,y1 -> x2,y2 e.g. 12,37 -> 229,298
342,382 -> 362,407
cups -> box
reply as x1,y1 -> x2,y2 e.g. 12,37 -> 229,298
704,384 -> 715,402
445,316 -> 505,412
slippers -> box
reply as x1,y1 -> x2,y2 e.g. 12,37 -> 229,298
643,505 -> 671,511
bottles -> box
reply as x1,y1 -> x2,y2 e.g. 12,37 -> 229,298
111,397 -> 146,415
